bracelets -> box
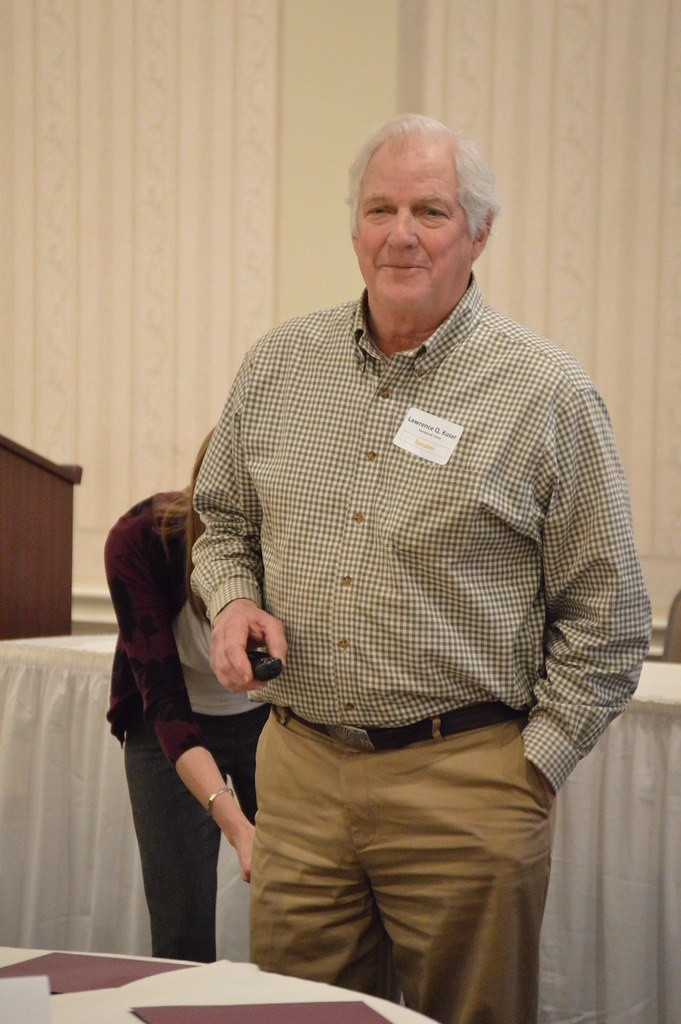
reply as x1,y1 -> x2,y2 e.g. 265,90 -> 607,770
208,788 -> 234,817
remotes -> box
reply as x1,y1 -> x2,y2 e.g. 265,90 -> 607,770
245,650 -> 283,682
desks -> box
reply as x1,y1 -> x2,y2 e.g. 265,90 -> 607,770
0,945 -> 436,1024
0,634 -> 681,1024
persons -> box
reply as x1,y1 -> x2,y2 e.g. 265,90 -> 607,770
104,429 -> 270,964
189,111 -> 652,1024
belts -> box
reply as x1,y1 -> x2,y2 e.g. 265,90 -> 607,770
285,700 -> 528,753
